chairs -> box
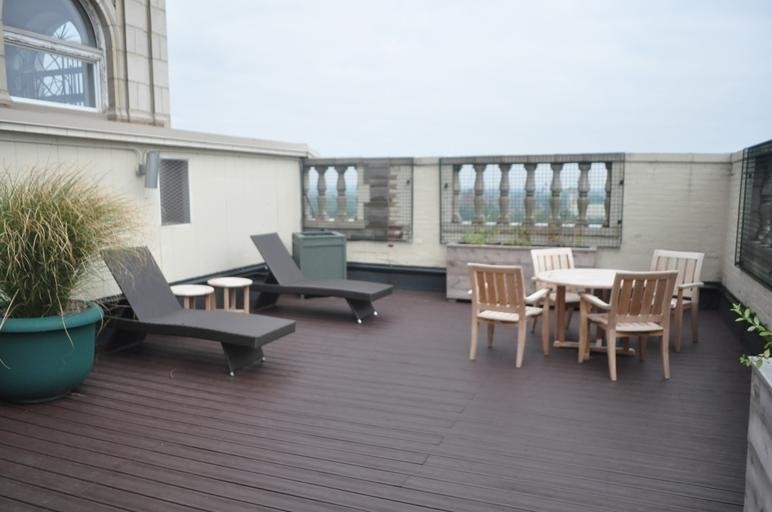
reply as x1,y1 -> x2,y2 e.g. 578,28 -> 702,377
578,269 -> 680,396
100,244 -> 297,377
466,262 -> 552,369
531,246 -> 591,336
250,231 -> 394,325
649,248 -> 705,353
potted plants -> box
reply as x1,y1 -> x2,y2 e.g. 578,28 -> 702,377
0,159 -> 166,405
446,224 -> 598,305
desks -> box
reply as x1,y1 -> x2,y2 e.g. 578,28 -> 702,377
532,268 -> 654,360
169,284 -> 214,311
207,277 -> 254,313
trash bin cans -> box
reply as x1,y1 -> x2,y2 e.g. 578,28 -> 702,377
293,231 -> 347,300
